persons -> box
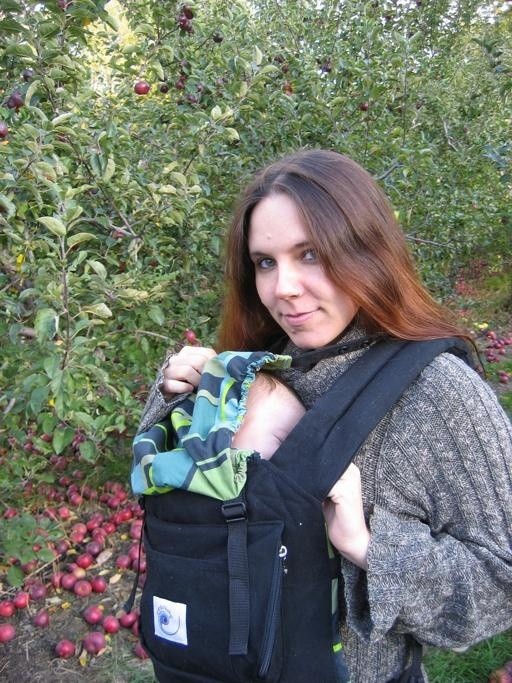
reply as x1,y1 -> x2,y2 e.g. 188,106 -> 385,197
231,370 -> 309,465
130,144 -> 511,682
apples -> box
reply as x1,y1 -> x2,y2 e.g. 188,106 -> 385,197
0,0 -> 512,682
